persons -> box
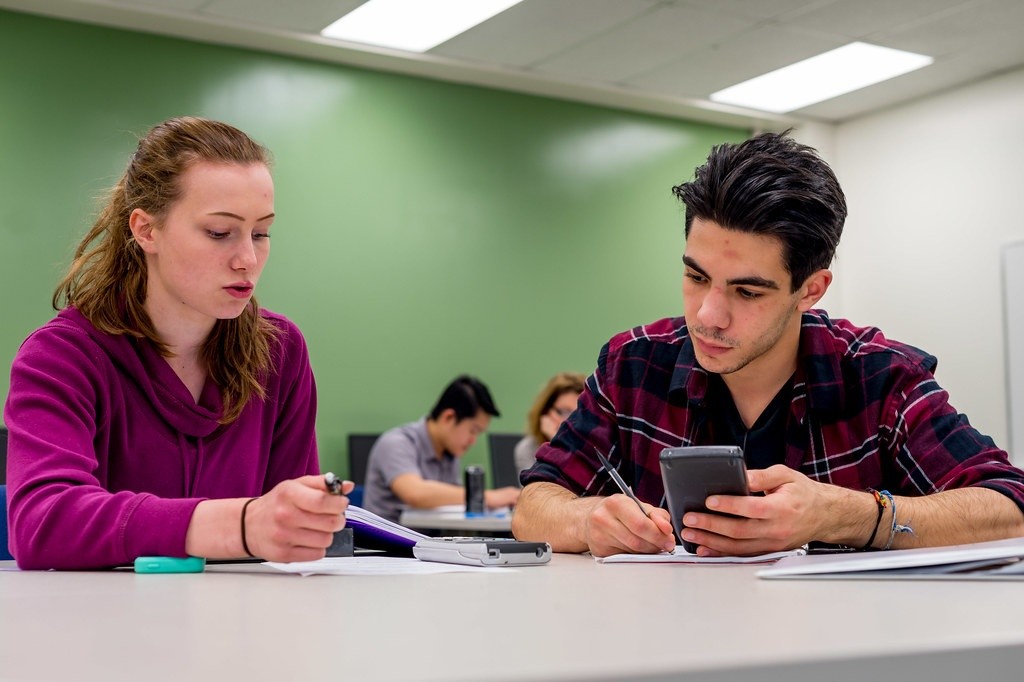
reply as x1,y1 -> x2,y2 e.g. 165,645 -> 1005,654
511,125 -> 1024,556
515,373 -> 586,488
4,116 -> 354,572
363,374 -> 520,526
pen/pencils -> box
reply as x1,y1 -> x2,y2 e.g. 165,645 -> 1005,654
323,470 -> 343,495
593,444 -> 677,555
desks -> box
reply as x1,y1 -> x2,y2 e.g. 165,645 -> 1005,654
0,507 -> 1024,682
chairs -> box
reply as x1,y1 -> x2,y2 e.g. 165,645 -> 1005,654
346,431 -> 383,488
487,433 -> 525,490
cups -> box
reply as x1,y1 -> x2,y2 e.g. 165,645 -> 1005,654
465,466 -> 485,514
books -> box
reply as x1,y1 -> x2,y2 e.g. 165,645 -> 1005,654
341,503 -> 433,554
600,544 -> 808,565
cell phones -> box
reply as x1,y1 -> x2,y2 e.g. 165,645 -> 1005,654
412,536 -> 552,566
133,556 -> 206,573
658,446 -> 753,554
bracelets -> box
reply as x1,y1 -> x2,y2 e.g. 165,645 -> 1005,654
880,490 -> 915,550
240,497 -> 257,558
856,488 -> 887,550
